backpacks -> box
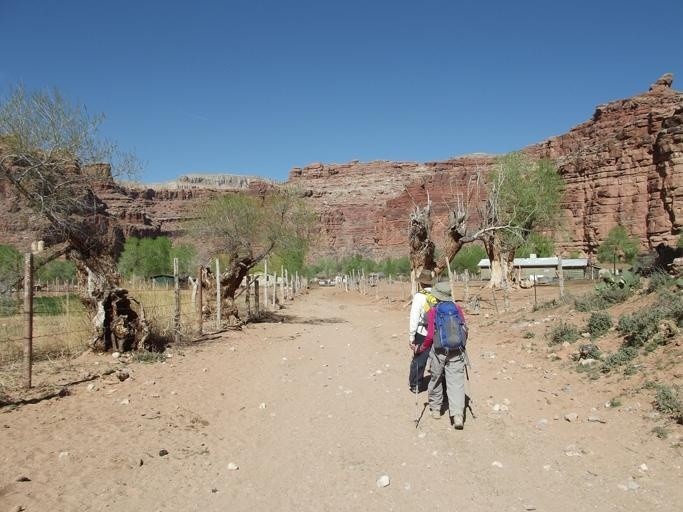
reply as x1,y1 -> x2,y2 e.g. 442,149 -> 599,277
418,290 -> 440,326
432,302 -> 467,356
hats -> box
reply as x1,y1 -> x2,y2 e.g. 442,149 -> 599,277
432,283 -> 453,301
415,269 -> 433,284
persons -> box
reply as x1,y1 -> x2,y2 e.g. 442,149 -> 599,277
408,269 -> 438,393
411,282 -> 468,429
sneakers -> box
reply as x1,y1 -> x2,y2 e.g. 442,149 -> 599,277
454,415 -> 464,429
428,411 -> 440,419
409,385 -> 419,393
